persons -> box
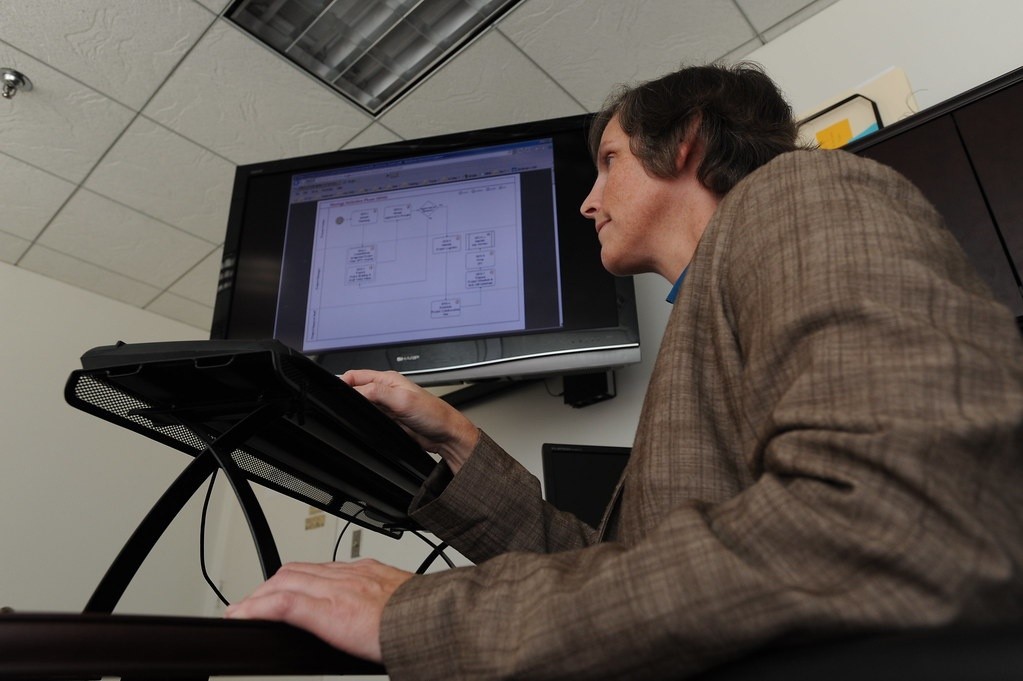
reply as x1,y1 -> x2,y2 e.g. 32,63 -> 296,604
222,62 -> 1023,681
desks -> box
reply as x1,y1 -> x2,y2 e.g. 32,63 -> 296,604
0,611 -> 387,681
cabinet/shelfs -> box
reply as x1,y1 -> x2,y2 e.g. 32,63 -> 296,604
833,66 -> 1023,331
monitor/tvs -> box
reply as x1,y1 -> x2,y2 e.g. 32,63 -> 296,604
542,443 -> 633,539
213,111 -> 641,384
